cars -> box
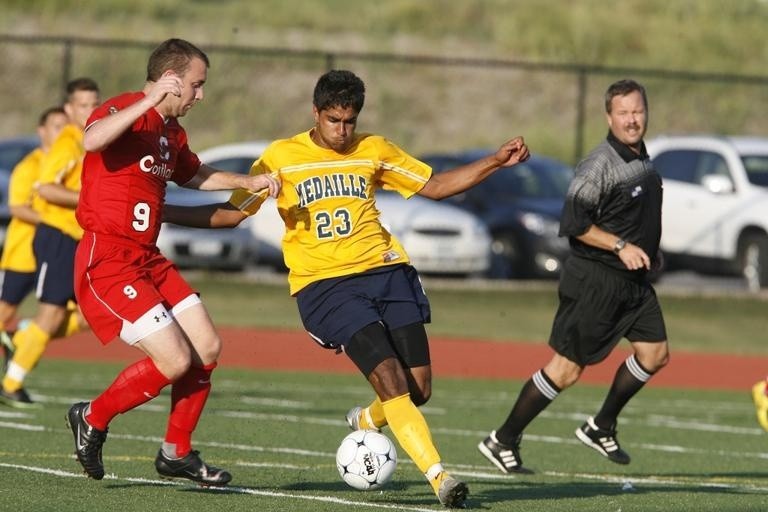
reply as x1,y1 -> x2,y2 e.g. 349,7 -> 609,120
0,133 -> 257,279
191,133 -> 492,279
408,151 -> 599,284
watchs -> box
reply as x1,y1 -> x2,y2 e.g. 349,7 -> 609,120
613,237 -> 630,254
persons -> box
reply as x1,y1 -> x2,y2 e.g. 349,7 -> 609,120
477,79 -> 666,476
66,36 -> 281,488
0,79 -> 103,412
1,105 -> 69,351
162,69 -> 532,511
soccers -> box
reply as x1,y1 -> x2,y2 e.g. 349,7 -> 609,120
336,430 -> 399,492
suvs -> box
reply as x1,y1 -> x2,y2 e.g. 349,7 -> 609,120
616,133 -> 768,296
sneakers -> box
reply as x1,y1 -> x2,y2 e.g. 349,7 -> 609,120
573,415 -> 632,466
154,447 -> 232,491
63,401 -> 108,481
0,384 -> 40,410
437,479 -> 470,509
476,431 -> 533,475
751,378 -> 768,434
344,406 -> 363,431
